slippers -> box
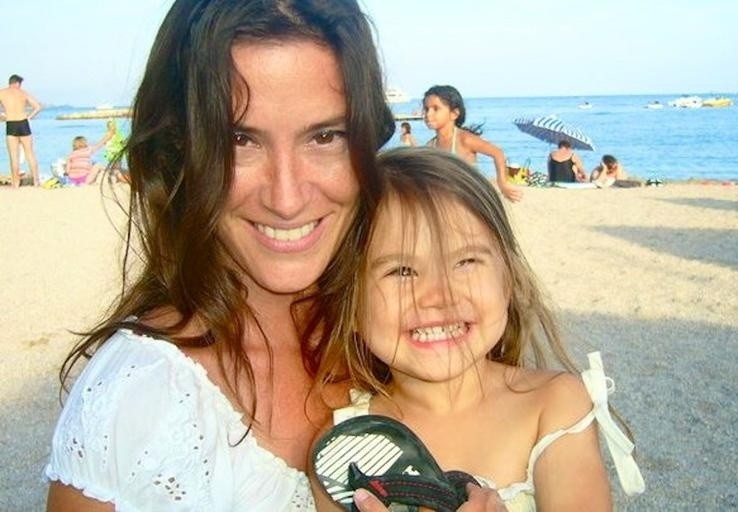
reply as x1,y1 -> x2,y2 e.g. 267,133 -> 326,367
309,415 -> 483,512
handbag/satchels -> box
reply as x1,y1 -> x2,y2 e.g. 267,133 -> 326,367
503,157 -> 531,185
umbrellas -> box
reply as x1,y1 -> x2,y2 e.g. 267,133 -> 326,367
512,115 -> 594,151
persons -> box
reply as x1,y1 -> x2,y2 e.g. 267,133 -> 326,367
52,129 -> 116,186
288,147 -> 646,512
42,0 -> 399,511
0,75 -> 42,187
399,122 -> 417,148
547,139 -> 587,184
589,154 -> 623,188
103,121 -> 129,182
422,85 -> 522,203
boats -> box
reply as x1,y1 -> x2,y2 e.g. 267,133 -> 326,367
387,88 -> 411,102
641,95 -> 734,109
395,114 -> 420,121
56,108 -> 132,120
